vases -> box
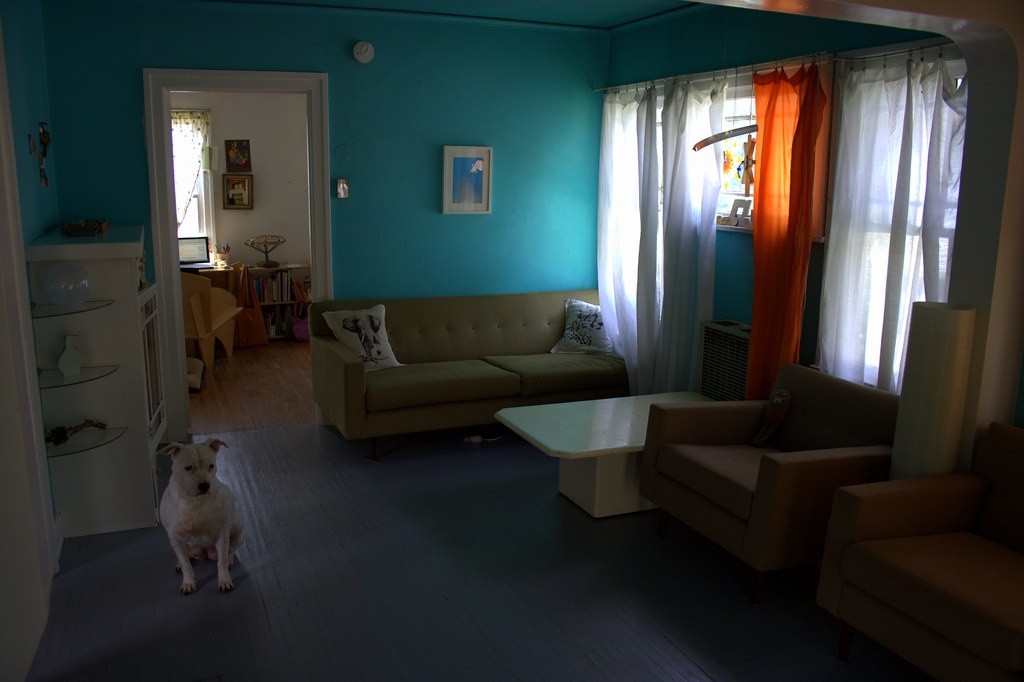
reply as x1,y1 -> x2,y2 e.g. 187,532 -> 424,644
216,254 -> 230,268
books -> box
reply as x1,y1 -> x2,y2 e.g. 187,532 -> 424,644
253,271 -> 306,336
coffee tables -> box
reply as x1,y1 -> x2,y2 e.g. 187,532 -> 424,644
494,392 -> 717,518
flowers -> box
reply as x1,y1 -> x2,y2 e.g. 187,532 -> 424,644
215,243 -> 231,254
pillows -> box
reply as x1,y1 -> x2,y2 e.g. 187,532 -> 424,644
550,297 -> 627,359
320,304 -> 405,372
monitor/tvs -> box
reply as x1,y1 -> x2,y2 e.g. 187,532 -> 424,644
177,237 -> 210,265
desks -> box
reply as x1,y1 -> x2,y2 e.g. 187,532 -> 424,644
199,257 -> 235,294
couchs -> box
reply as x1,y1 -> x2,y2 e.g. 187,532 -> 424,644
817,420 -> 1024,682
638,362 -> 901,607
307,290 -> 630,459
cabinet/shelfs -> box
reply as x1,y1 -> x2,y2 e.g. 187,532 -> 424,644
25,228 -> 165,537
248,264 -> 311,339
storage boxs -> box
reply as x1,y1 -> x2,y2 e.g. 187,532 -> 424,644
60,220 -> 108,237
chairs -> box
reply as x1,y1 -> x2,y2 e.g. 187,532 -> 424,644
181,272 -> 242,368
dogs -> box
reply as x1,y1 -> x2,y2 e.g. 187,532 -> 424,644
155,438 -> 245,596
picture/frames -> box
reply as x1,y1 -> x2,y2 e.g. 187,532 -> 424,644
222,173 -> 253,210
439,146 -> 493,215
